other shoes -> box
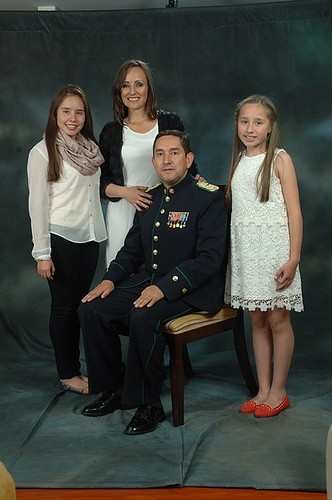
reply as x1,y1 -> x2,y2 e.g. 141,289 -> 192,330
254,395 -> 290,417
60,374 -> 89,394
239,400 -> 260,414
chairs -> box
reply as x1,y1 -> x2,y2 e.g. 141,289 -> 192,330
119,185 -> 259,428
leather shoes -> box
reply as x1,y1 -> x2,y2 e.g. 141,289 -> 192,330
82,390 -> 122,416
125,399 -> 165,434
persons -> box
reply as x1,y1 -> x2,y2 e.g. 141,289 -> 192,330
224,94 -> 306,417
27,84 -> 108,395
80,129 -> 228,436
98,60 -> 207,274
